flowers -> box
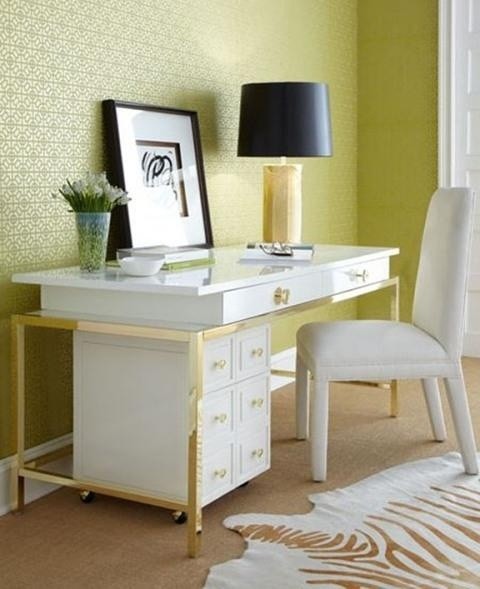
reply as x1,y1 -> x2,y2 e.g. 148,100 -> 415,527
51,173 -> 132,270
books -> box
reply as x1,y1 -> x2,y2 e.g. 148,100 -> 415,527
236,240 -> 316,262
132,243 -> 216,272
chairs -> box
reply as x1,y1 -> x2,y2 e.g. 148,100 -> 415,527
295,187 -> 478,483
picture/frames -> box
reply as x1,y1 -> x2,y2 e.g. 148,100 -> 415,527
103,99 -> 215,251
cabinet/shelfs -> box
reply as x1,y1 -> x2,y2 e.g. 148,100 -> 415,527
74,323 -> 273,524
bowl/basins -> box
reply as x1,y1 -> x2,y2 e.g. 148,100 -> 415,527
118,256 -> 164,276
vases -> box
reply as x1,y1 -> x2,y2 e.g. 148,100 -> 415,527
75,211 -> 112,270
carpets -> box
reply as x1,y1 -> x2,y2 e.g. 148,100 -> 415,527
203,451 -> 480,589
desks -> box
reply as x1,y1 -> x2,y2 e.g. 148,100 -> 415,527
9,243 -> 400,558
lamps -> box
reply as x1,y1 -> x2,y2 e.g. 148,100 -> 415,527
237,82 -> 332,245
